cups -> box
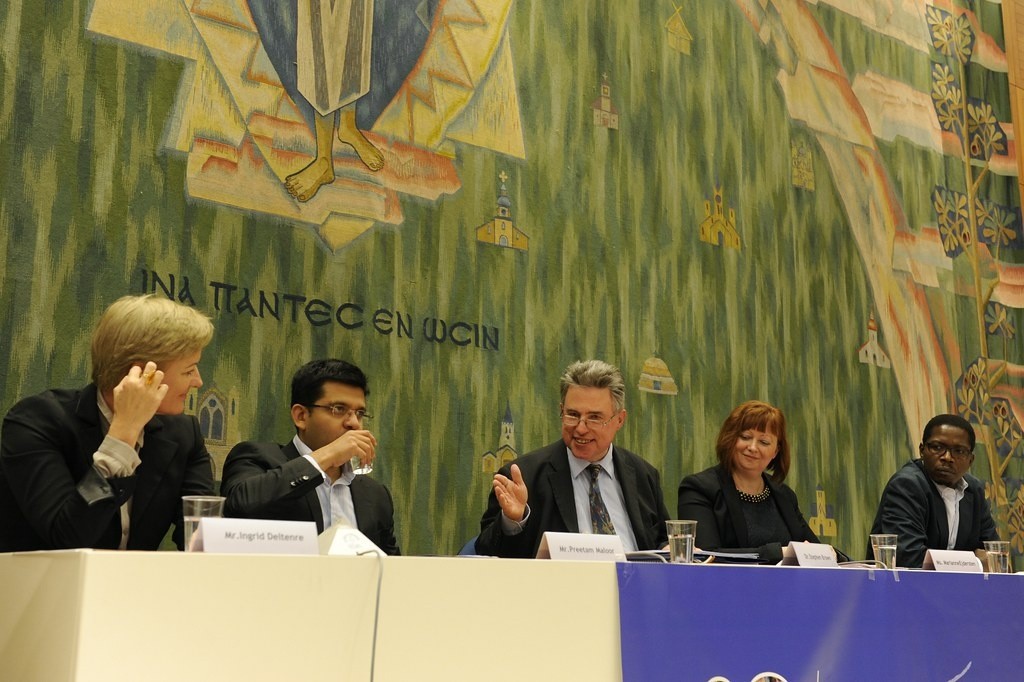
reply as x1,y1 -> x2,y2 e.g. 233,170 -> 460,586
181,496 -> 227,552
350,456 -> 373,474
665,520 -> 698,563
870,534 -> 898,569
983,541 -> 1010,573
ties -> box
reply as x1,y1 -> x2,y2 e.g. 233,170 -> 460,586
585,464 -> 617,535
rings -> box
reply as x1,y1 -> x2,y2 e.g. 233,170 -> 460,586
140,373 -> 152,379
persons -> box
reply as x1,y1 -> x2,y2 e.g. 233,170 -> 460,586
677,400 -> 856,566
866,414 -> 1012,572
219,358 -> 402,556
0,292 -> 221,550
474,359 -> 682,558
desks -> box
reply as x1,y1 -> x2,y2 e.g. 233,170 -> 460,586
0,548 -> 1024,682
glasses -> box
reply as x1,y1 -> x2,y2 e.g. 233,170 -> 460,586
559,410 -> 616,430
924,439 -> 972,460
302,403 -> 373,426
